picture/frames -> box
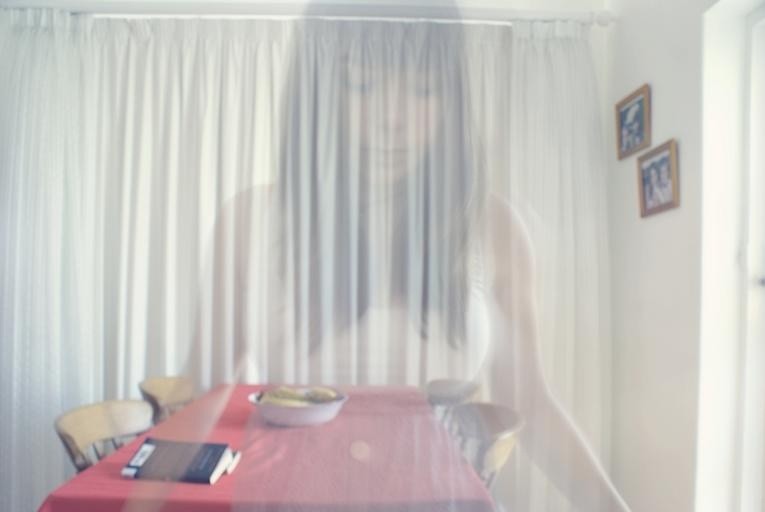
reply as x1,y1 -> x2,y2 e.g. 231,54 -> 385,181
615,83 -> 679,218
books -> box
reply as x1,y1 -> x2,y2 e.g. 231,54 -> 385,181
121,437 -> 241,484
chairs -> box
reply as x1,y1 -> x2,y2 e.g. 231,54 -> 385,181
445,397 -> 523,491
421,376 -> 484,437
138,374 -> 196,424
56,398 -> 156,473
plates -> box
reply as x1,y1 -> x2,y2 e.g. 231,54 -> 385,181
247,388 -> 348,428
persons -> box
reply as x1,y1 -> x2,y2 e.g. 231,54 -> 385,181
642,157 -> 672,210
178,1 -> 631,512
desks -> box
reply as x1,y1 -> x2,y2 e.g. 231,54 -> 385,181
46,384 -> 497,511
